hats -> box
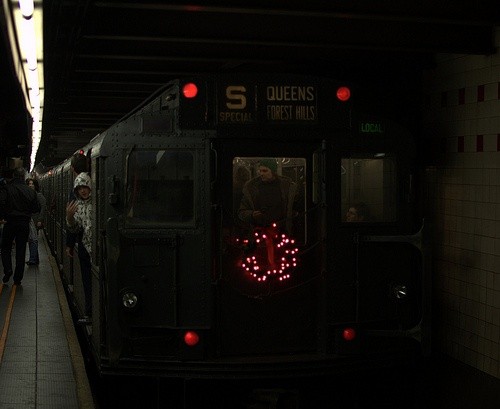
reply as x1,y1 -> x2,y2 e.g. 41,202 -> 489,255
258,158 -> 278,176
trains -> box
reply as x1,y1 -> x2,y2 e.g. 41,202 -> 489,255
36,63 -> 445,407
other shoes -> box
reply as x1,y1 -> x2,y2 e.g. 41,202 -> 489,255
26,261 -> 39,265
2,270 -> 13,283
14,281 -> 21,286
78,315 -> 92,323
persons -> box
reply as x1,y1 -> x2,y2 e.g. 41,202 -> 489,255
64,153 -> 92,327
0,166 -> 47,286
345,201 -> 371,225
237,160 -> 305,270
